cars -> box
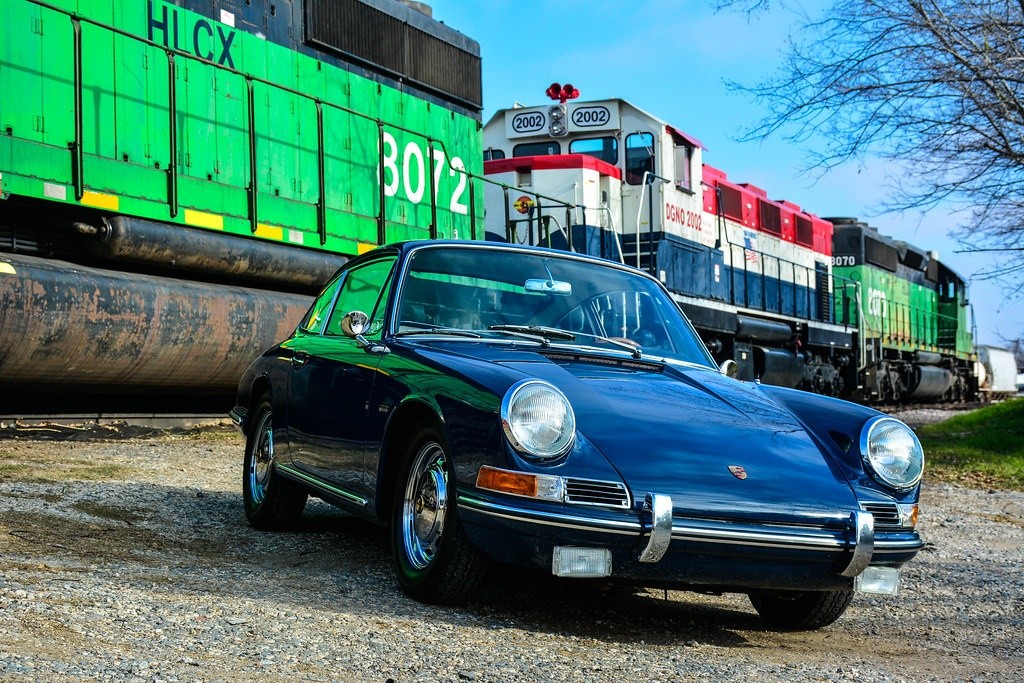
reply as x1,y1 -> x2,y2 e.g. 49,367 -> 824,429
225,238 -> 926,632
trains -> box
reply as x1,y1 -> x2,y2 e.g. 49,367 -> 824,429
0,1 -> 992,414
